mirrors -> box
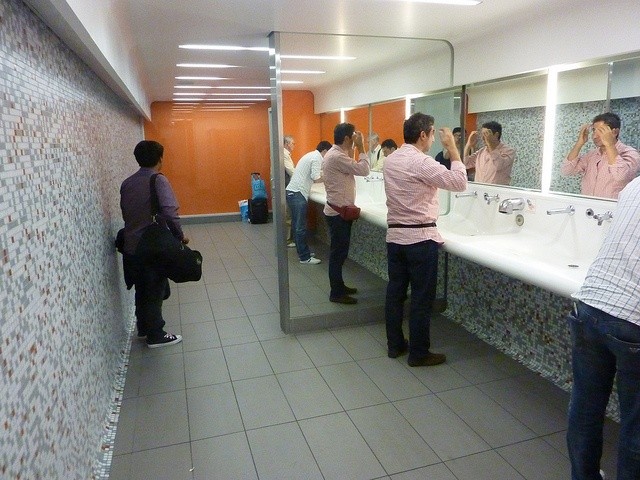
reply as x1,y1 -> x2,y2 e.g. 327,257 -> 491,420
369,100 -> 406,166
337,105 -> 370,163
268,31 -> 454,331
319,112 -> 340,147
408,95 -> 462,216
547,48 -> 640,203
462,71 -> 544,191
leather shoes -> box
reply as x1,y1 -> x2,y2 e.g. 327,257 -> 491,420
388,339 -> 408,358
330,294 -> 357,304
345,286 -> 357,294
408,352 -> 445,367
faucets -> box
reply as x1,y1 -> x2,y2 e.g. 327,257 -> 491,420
483,192 -> 500,205
593,211 -> 614,226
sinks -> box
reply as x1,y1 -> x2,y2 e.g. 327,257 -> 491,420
533,241 -> 595,278
453,218 -> 521,235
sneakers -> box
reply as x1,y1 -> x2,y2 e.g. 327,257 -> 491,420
136,334 -> 147,339
600,469 -> 605,479
287,242 -> 296,247
310,252 -> 315,257
146,333 -> 182,349
300,257 -> 321,264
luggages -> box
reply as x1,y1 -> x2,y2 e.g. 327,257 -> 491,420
249,172 -> 268,224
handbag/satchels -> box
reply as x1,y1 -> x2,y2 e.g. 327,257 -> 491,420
136,222 -> 202,282
238,199 -> 249,222
251,175 -> 268,200
327,201 -> 360,220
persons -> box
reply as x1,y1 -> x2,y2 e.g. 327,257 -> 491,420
283,135 -> 296,247
383,112 -> 468,366
464,122 -> 515,185
435,127 -> 471,180
366,133 -> 382,170
373,139 -> 400,172
120,141 -> 189,349
285,141 -> 332,264
560,112 -> 640,200
565,176 -> 640,479
321,123 -> 370,304
